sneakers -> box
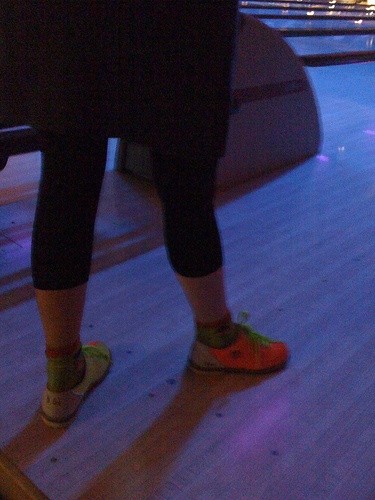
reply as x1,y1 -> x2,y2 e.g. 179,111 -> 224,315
40,341 -> 113,430
187,323 -> 289,377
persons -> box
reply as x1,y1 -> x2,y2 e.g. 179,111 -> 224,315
1,0 -> 291,428
